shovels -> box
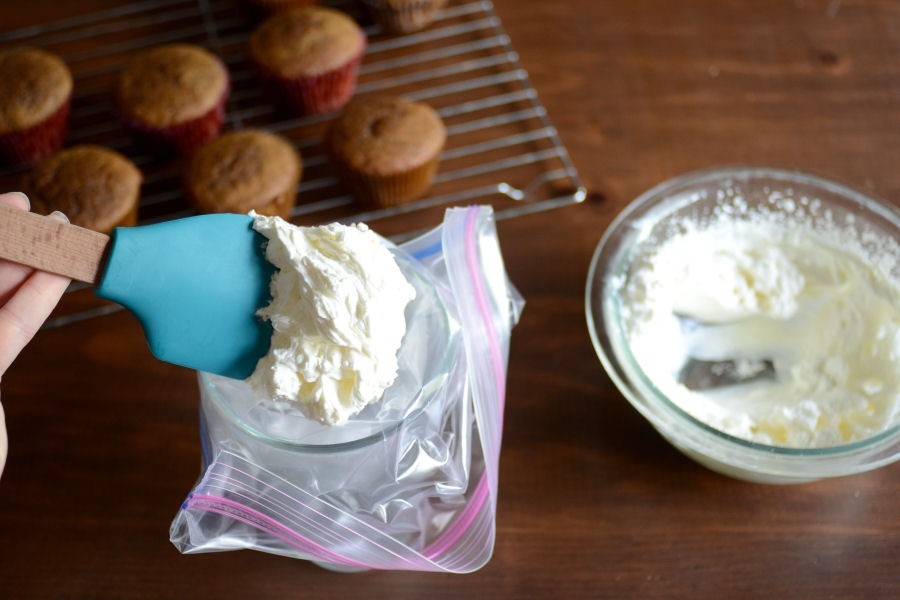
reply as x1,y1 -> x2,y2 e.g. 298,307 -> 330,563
1,206 -> 279,380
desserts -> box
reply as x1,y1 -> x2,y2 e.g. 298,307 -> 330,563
17,144 -> 143,235
0,41 -> 75,178
113,44 -> 229,154
333,95 -> 447,206
182,129 -> 303,220
370,0 -> 446,34
249,6 -> 366,115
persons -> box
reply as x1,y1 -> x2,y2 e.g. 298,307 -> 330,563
0,192 -> 72,479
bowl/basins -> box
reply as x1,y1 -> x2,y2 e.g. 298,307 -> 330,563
584,163 -> 900,485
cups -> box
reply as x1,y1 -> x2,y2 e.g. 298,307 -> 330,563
198,227 -> 455,573
